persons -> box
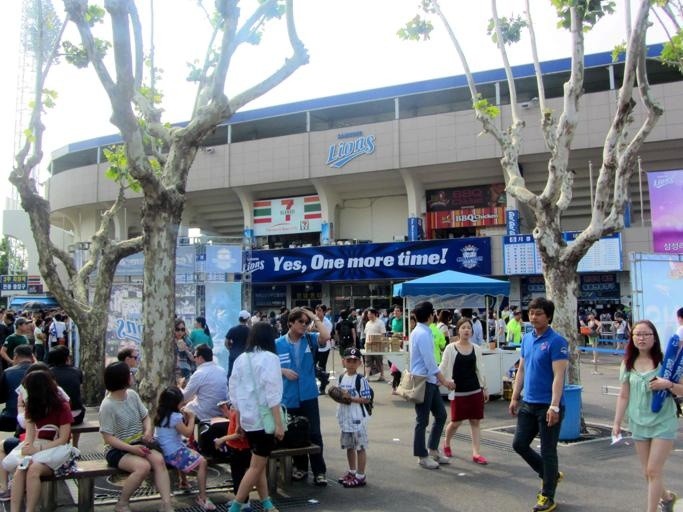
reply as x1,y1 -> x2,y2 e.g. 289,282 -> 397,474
610,321 -> 683,512
457,308 -> 483,346
152,385 -> 218,511
436,309 -> 452,351
508,296 -> 563,512
333,304 -> 367,353
336,347 -> 374,488
578,305 -> 630,363
214,401 -> 252,510
179,344 -> 230,425
117,348 -> 140,390
0,344 -> 38,432
390,306 -> 409,336
229,322 -> 285,512
0,362 -> 70,501
188,316 -> 214,359
671,307 -> 683,357
174,319 -> 197,388
405,302 -> 451,470
98,360 -> 174,512
387,340 -> 405,394
0,299 -> 74,362
491,303 -> 522,346
45,344 -> 86,448
430,315 -> 447,370
364,310 -> 386,381
309,304 -> 334,395
276,307 -> 330,487
225,310 -> 252,386
438,317 -> 491,465
410,315 -> 417,332
10,369 -> 74,512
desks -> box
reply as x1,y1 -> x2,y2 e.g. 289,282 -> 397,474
358,345 -> 406,381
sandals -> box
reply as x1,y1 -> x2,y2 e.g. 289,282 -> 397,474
338,471 -> 356,484
342,474 -> 367,487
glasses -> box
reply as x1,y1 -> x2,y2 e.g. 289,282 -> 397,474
175,327 -> 185,332
194,356 -> 199,358
130,356 -> 137,359
632,332 -> 654,337
297,319 -> 309,325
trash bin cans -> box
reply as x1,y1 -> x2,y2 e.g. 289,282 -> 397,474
558,383 -> 584,441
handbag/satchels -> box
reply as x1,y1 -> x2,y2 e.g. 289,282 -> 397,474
395,368 -> 428,404
53,323 -> 65,345
246,350 -> 288,434
594,319 -> 602,339
32,424 -> 60,446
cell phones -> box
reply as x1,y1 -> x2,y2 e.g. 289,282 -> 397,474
647,376 -> 657,382
142,446 -> 152,454
19,456 -> 33,470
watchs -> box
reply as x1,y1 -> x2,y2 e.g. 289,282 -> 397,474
550,406 -> 560,413
668,383 -> 674,391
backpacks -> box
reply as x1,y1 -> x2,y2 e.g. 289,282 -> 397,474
340,371 -> 374,417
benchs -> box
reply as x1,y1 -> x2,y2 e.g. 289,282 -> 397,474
266,434 -> 323,503
10,441 -> 272,512
68,416 -> 105,452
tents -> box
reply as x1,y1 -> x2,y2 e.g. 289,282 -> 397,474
393,270 -> 511,354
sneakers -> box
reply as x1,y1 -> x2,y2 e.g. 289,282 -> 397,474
532,496 -> 557,512
0,489 -> 10,501
264,506 -> 279,512
292,468 -> 308,480
659,489 -> 677,512
443,443 -> 452,457
472,455 -> 487,464
314,473 -> 328,484
419,456 -> 439,469
536,470 -> 563,501
225,500 -> 250,506
228,502 -> 252,512
427,448 -> 449,464
195,495 -> 217,512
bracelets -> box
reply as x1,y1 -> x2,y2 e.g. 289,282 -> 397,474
40,444 -> 43,452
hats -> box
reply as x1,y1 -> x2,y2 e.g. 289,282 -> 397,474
16,317 -> 32,326
239,310 -> 250,320
344,347 -> 362,360
514,310 -> 522,315
